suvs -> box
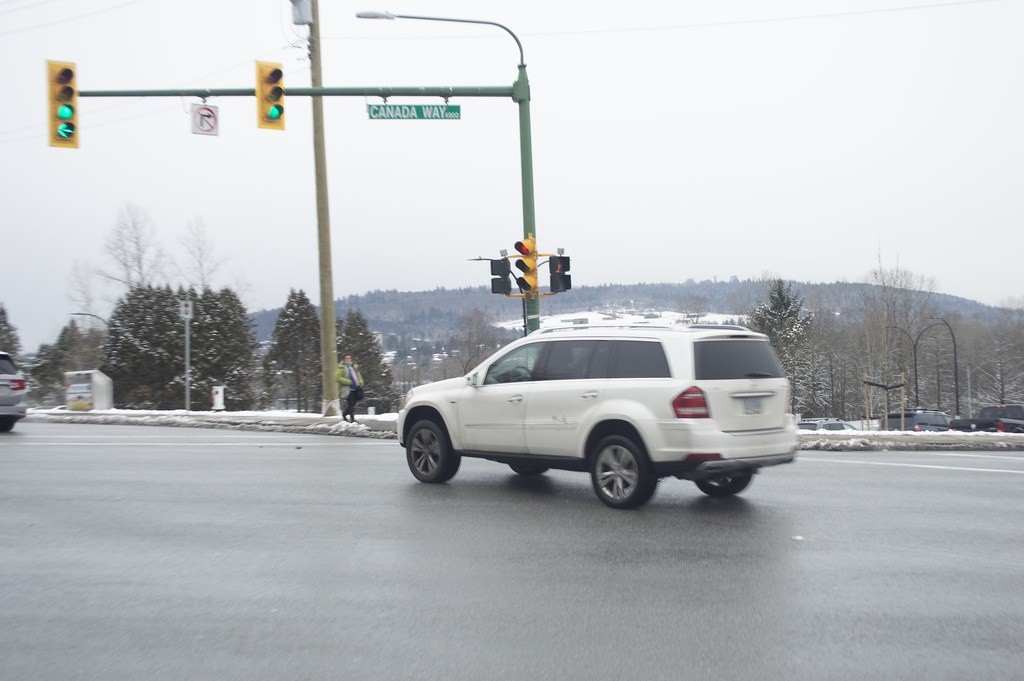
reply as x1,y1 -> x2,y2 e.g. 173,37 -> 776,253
798,418 -> 858,431
878,398 -> 1024,433
0,351 -> 28,433
396,321 -> 795,510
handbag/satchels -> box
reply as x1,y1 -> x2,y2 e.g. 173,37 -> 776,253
355,386 -> 364,400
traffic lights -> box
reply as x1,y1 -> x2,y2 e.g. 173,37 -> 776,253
514,238 -> 538,293
550,256 -> 573,293
46,59 -> 78,148
490,258 -> 511,296
255,60 -> 285,130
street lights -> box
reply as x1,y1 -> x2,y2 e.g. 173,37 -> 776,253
884,324 -> 944,407
832,309 -> 874,418
861,381 -> 905,428
930,316 -> 959,416
357,11 -> 539,366
468,255 -> 527,365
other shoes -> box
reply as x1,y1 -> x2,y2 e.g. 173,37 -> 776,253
351,420 -> 359,423
343,412 -> 347,421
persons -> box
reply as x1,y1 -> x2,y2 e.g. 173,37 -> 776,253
335,354 -> 364,422
